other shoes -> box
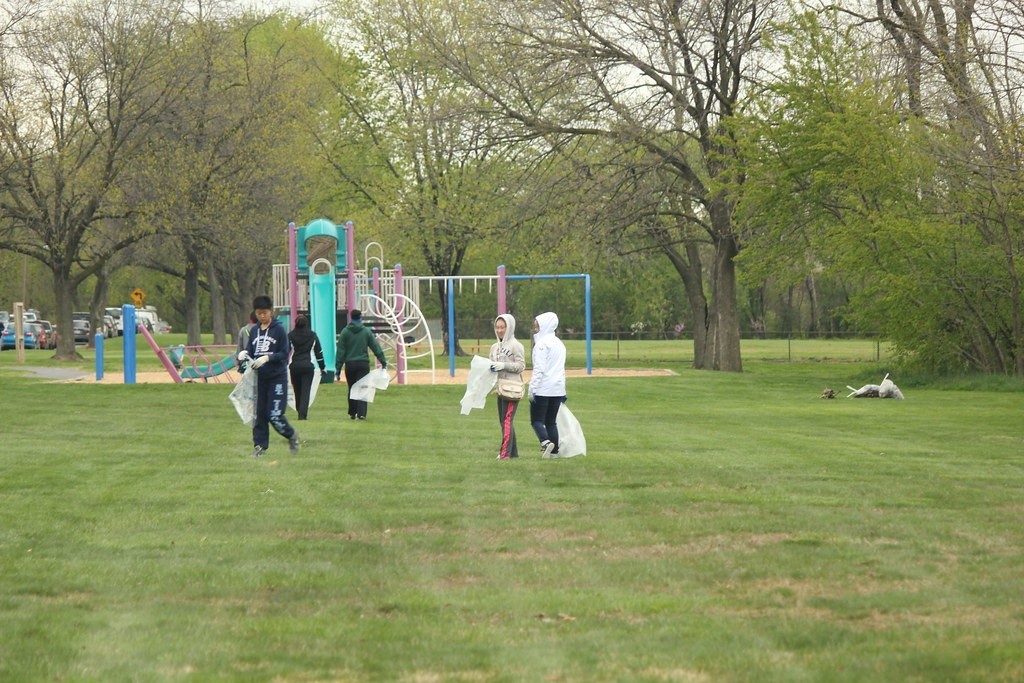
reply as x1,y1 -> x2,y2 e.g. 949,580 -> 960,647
350,414 -> 365,419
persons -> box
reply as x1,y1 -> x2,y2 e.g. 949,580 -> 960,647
487,313 -> 528,459
529,311 -> 570,459
334,309 -> 388,421
235,295 -> 301,458
289,315 -> 327,421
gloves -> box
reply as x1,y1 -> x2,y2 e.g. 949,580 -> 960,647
490,362 -> 505,372
251,355 -> 269,369
336,371 -> 340,382
562,396 -> 567,403
321,367 -> 327,380
237,349 -> 248,361
528,389 -> 536,405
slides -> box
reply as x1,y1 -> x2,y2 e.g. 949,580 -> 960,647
180,307 -> 289,379
307,259 -> 339,381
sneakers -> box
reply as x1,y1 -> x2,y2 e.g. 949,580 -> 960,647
539,441 -> 558,459
288,430 -> 300,457
249,445 -> 266,458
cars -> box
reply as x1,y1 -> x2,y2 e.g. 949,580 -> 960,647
0,304 -> 172,349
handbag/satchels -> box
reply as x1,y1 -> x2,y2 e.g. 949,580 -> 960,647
497,378 -> 526,401
